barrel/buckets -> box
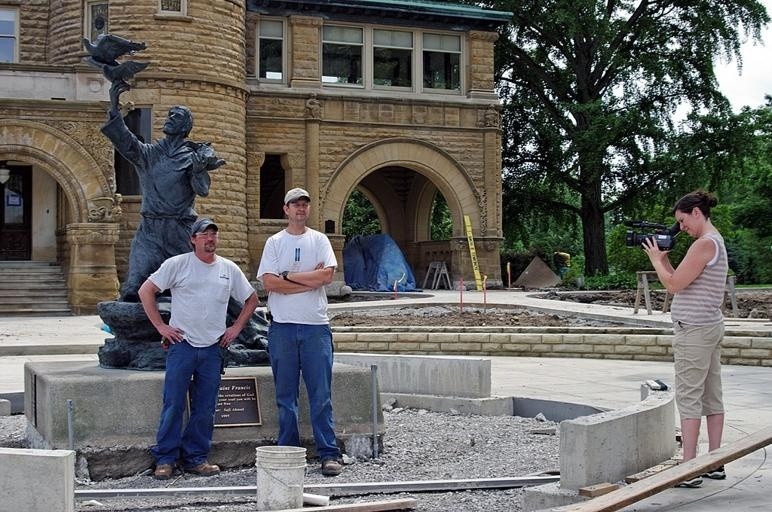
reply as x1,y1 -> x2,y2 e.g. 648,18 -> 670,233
254,446 -> 307,511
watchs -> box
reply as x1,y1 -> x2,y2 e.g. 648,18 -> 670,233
282,271 -> 289,279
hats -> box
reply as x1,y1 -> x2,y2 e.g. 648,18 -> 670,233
284,188 -> 311,205
192,218 -> 218,235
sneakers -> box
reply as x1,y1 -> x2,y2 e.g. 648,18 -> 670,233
322,460 -> 342,475
672,475 -> 703,488
700,466 -> 726,479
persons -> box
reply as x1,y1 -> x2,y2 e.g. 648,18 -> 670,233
641,189 -> 729,485
137,218 -> 259,480
102,82 -> 215,301
255,186 -> 342,477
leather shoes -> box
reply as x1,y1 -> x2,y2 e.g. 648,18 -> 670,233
184,462 -> 220,476
155,464 -> 172,480
